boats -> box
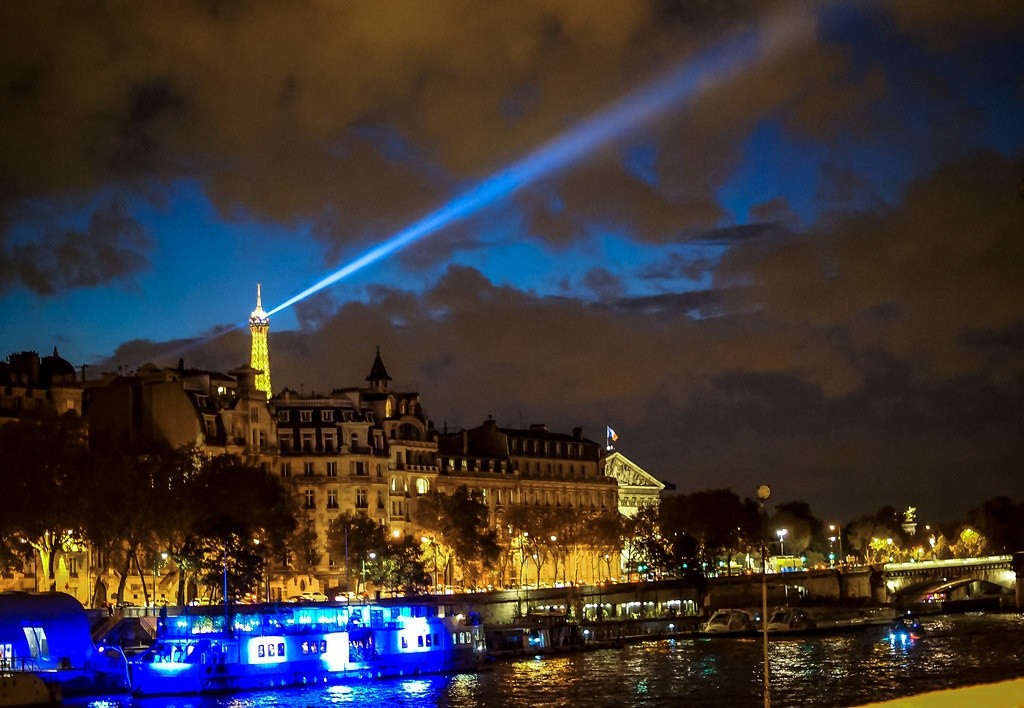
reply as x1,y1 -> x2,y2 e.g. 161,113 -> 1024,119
93,602 -> 458,695
698,599 -> 912,637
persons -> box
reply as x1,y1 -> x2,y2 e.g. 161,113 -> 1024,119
108,603 -> 114,618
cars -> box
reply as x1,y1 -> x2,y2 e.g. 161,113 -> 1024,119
234,593 -> 267,606
143,598 -> 178,608
188,597 -> 219,607
288,595 -> 310,603
333,579 -> 588,602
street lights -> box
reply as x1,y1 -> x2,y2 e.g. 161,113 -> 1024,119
829,524 -> 843,574
421,537 -> 438,596
524,532 -> 528,586
776,528 -> 788,557
755,484 -> 773,708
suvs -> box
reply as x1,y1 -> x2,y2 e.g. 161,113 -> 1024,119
303,591 -> 329,602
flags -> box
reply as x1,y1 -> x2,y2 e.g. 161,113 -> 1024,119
607,426 -> 618,441
